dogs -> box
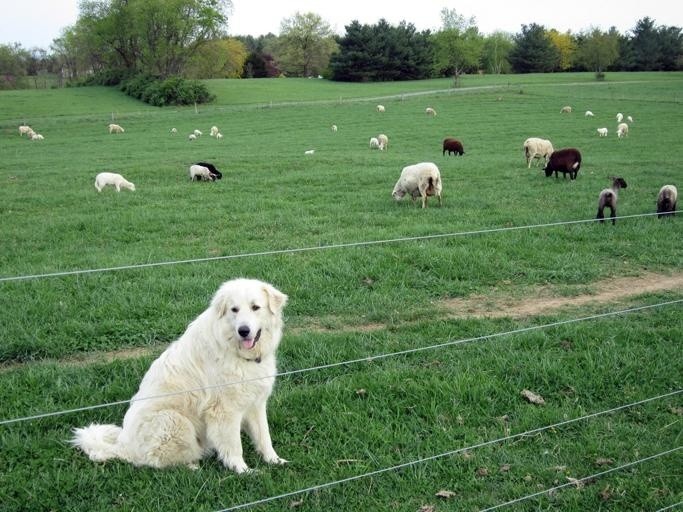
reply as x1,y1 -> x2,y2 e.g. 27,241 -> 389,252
62,278 -> 294,476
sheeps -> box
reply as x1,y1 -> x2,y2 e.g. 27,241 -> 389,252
392,162 -> 443,208
18,125 -> 43,141
189,162 -> 222,182
188,126 -> 224,140
596,112 -> 633,138
426,108 -> 436,117
523,138 -> 582,180
370,133 -> 388,152
560,106 -> 572,114
95,173 -> 135,192
655,184 -> 678,219
108,124 -> 126,135
585,111 -> 594,116
442,138 -> 466,156
376,105 -> 385,112
591,175 -> 628,225
